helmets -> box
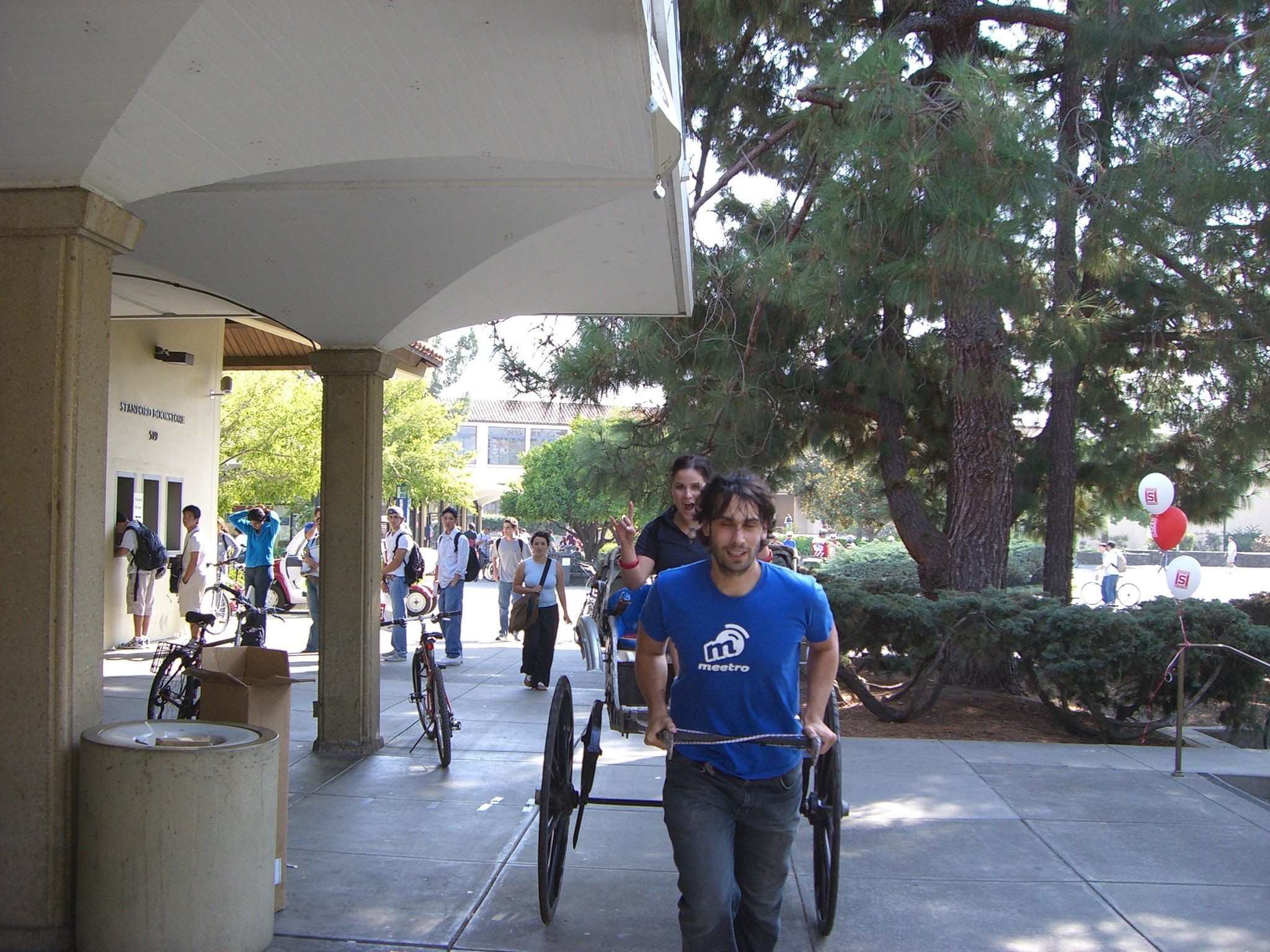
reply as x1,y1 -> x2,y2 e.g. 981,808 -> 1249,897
847,535 -> 854,542
829,534 -> 838,541
786,531 -> 793,536
819,529 -> 828,534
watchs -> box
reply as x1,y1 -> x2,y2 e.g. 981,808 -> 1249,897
380,580 -> 385,584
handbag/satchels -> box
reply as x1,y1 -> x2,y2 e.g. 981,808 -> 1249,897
170,567 -> 184,593
300,562 -> 313,577
508,593 -> 539,634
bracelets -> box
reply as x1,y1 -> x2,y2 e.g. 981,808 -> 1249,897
619,555 -> 639,570
761,549 -> 774,562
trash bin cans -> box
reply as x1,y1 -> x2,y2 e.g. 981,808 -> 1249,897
74,718 -> 278,952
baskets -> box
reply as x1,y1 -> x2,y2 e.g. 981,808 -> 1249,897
150,641 -> 182,676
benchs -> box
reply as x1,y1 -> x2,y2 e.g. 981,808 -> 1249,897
600,540 -> 805,708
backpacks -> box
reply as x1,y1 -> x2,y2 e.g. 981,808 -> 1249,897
122,519 -> 167,571
787,517 -> 791,522
440,532 -> 480,582
1111,555 -> 1126,572
387,531 -> 425,587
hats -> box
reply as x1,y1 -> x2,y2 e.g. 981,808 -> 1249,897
387,506 -> 406,521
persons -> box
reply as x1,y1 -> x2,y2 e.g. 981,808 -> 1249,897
462,526 -> 584,580
513,531 -> 573,691
1223,534 -> 1239,574
783,514 -> 793,528
491,518 -> 533,641
293,504 -> 421,664
175,504 -> 207,650
433,507 -> 467,666
606,454 -> 772,708
633,466 -> 840,952
1096,542 -> 1127,610
228,505 -> 281,645
812,528 -> 856,560
115,511 -> 157,649
770,535 -> 781,545
782,530 -> 796,549
232,526 -> 250,570
214,516 -> 239,617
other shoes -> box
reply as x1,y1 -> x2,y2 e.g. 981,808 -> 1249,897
180,639 -> 199,654
496,634 -> 507,642
301,650 -> 309,653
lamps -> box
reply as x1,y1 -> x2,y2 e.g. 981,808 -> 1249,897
154,350 -> 195,367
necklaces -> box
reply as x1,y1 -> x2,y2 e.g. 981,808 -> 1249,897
680,515 -> 702,540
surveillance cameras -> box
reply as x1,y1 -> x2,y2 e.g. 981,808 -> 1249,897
222,376 -> 232,395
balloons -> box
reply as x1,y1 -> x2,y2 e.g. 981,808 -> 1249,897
1138,473 -> 1202,601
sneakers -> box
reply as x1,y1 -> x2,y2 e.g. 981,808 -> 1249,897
382,651 -> 395,656
435,655 -> 461,666
385,654 -> 407,662
460,655 -> 463,663
120,637 -> 149,649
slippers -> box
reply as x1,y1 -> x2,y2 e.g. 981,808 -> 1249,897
524,674 -> 532,687
536,682 -> 547,691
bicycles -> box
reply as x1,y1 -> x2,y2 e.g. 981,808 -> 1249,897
196,557 -> 253,635
146,583 -> 287,720
1080,569 -> 1141,608
379,610 -> 464,767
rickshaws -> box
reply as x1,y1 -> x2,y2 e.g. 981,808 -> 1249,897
528,540 -> 854,935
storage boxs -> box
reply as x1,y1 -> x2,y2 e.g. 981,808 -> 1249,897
180,644 -> 317,912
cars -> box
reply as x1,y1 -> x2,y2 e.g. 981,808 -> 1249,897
264,515 -> 437,617
